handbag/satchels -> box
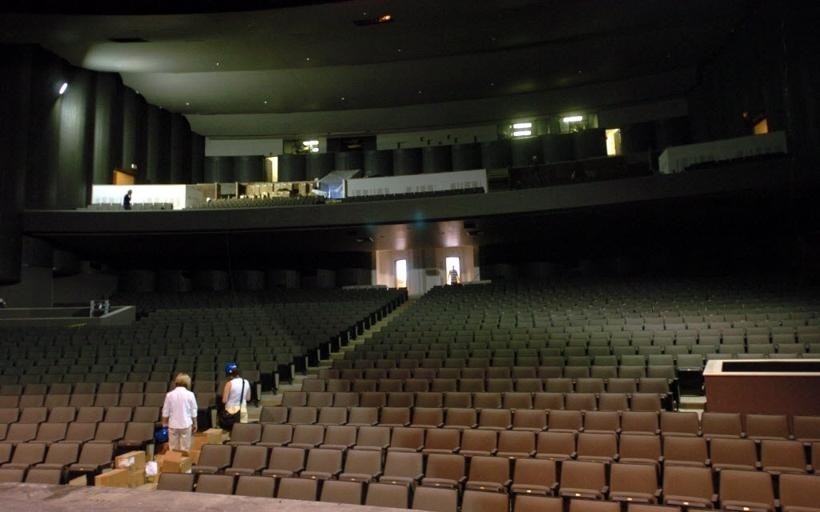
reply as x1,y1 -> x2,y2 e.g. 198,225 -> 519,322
220,409 -> 240,431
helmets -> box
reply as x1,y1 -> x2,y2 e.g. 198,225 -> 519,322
224,362 -> 237,376
155,427 -> 168,443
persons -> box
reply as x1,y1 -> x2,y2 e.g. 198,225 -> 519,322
159,371 -> 201,460
219,359 -> 253,443
121,189 -> 133,211
447,266 -> 461,284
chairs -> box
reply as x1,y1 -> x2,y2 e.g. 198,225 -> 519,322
1,280 -> 819,512
77,154 -> 646,214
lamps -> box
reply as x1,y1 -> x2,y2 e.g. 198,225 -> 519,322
44,73 -> 69,96
